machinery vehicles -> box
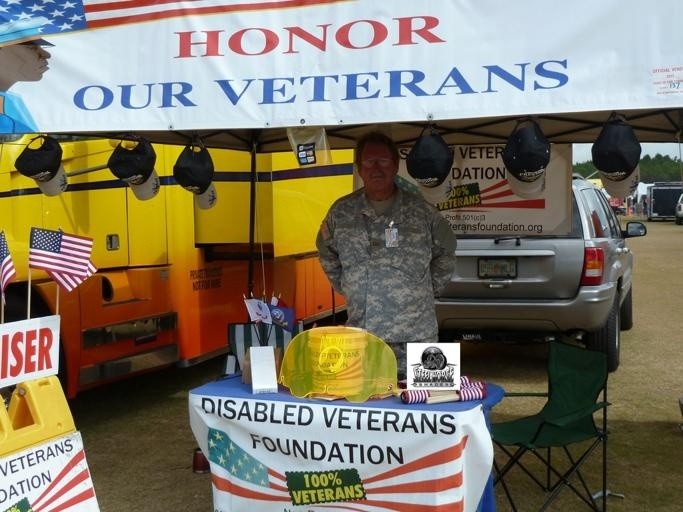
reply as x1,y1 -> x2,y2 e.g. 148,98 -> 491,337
1,132 -> 356,397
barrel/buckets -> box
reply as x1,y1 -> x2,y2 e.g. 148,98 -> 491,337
311,328 -> 368,398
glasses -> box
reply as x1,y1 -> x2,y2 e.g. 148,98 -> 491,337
358,156 -> 393,170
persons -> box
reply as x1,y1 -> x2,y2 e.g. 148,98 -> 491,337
0,39 -> 56,133
316,132 -> 457,381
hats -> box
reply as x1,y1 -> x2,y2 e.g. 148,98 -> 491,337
406,124 -> 454,204
591,113 -> 641,199
14,136 -> 69,196
173,142 -> 218,209
501,119 -> 551,199
108,135 -> 161,201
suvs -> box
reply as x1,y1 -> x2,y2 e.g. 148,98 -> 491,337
675,193 -> 683,224
436,170 -> 647,371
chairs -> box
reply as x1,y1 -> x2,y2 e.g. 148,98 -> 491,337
489,335 -> 614,510
222,322 -> 307,374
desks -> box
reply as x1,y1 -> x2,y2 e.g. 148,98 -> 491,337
187,370 -> 518,511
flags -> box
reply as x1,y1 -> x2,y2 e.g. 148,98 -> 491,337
0,232 -> 16,305
46,259 -> 98,295
0,0 -> 358,49
244,297 -> 295,332
29,227 -> 93,277
